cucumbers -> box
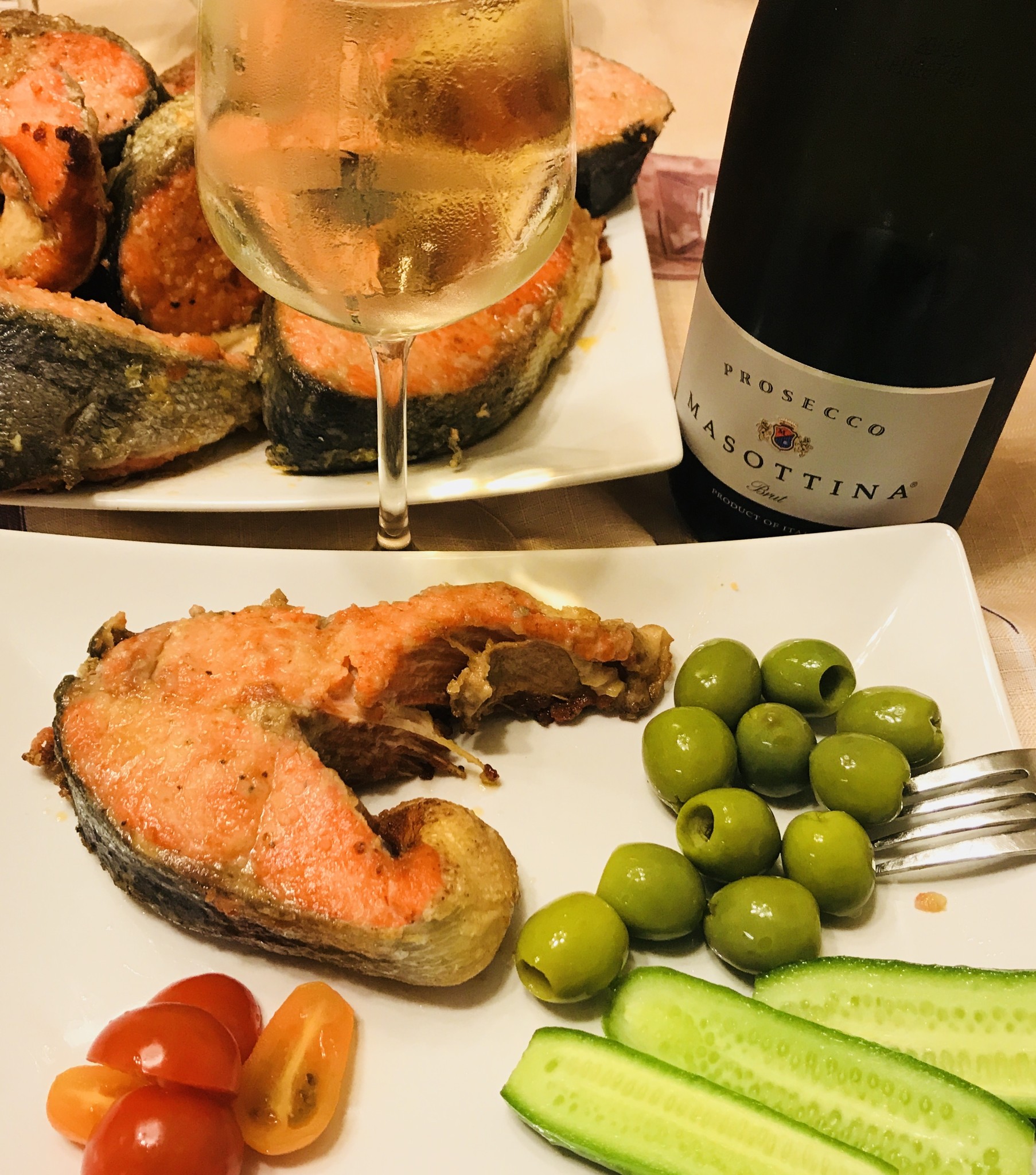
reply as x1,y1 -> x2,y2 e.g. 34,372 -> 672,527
751,955 -> 1036,1121
601,966 -> 1036,1175
500,1026 -> 901,1175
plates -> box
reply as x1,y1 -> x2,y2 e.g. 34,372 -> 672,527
0,185 -> 685,513
2,522 -> 1036,1175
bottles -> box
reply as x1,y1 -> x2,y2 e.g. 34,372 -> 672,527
671,1 -> 1036,541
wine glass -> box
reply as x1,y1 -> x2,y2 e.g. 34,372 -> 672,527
191,2 -> 579,553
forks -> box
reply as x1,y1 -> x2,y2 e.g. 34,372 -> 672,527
868,749 -> 1035,878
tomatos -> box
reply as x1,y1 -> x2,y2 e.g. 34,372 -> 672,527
42,973 -> 358,1175
